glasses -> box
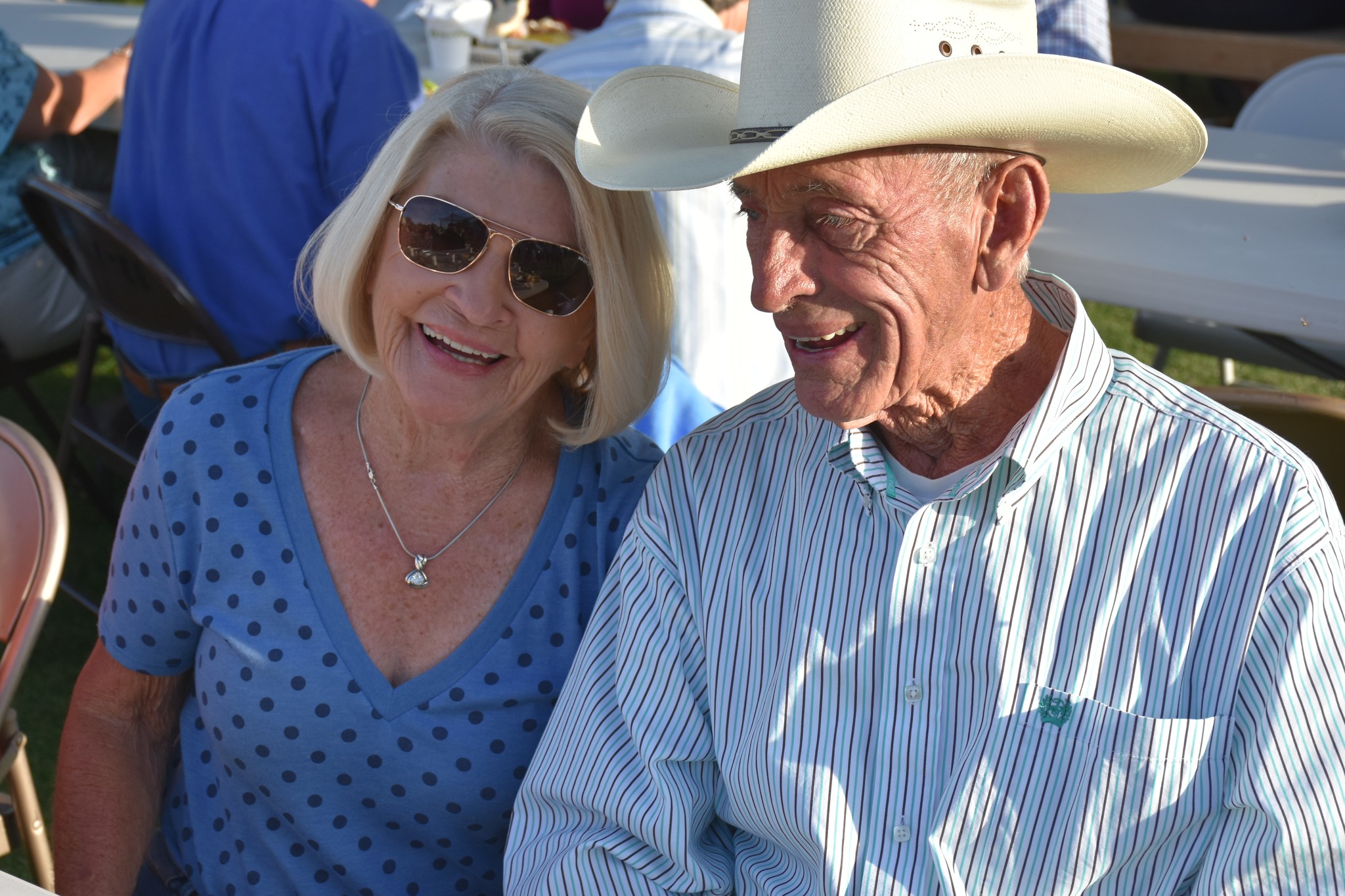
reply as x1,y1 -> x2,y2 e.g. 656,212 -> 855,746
388,193 -> 596,317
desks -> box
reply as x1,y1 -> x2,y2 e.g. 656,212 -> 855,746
1030,127 -> 1345,346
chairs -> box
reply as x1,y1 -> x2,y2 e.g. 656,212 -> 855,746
17,176 -> 228,613
0,416 -> 70,893
1135,54 -> 1345,381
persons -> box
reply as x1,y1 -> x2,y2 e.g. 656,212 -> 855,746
0,0 -> 1113,458
52,63 -> 667,896
503,0 -> 1345,896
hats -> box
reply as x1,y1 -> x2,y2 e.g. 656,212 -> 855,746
574,0 -> 1207,199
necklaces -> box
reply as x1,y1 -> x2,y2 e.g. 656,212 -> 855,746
356,373 -> 542,588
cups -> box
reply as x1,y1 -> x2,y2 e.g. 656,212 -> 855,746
423,17 -> 472,84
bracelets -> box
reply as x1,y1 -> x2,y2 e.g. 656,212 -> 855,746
112,48 -> 133,59
